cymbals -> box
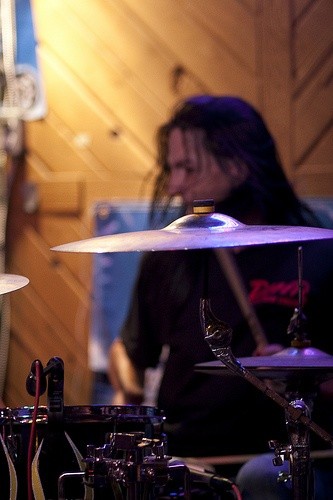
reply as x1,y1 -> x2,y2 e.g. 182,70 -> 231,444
0,272 -> 29,296
51,213 -> 333,252
195,348 -> 333,368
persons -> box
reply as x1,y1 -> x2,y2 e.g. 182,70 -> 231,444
109,94 -> 333,455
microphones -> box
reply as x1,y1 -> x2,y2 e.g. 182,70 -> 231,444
26,359 -> 47,397
190,470 -> 233,491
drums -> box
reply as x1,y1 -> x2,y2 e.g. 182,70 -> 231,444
0,405 -> 164,500
